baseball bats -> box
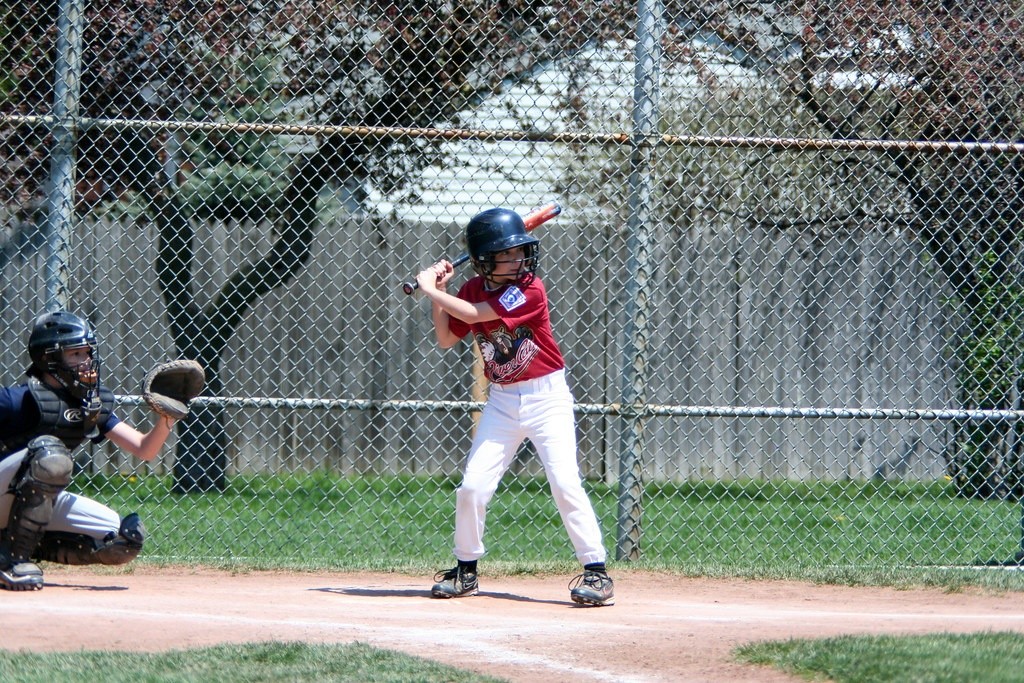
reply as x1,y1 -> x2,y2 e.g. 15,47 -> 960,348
402,202 -> 561,296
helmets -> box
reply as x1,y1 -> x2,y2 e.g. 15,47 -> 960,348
466,208 -> 540,285
28,310 -> 101,392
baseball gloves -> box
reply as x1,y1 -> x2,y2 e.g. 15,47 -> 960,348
142,360 -> 205,417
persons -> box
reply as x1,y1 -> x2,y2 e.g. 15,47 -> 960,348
0,310 -> 205,591
416,208 -> 615,607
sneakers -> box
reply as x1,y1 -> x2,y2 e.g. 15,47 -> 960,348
570,570 -> 616,606
431,568 -> 480,598
0,553 -> 48,592
